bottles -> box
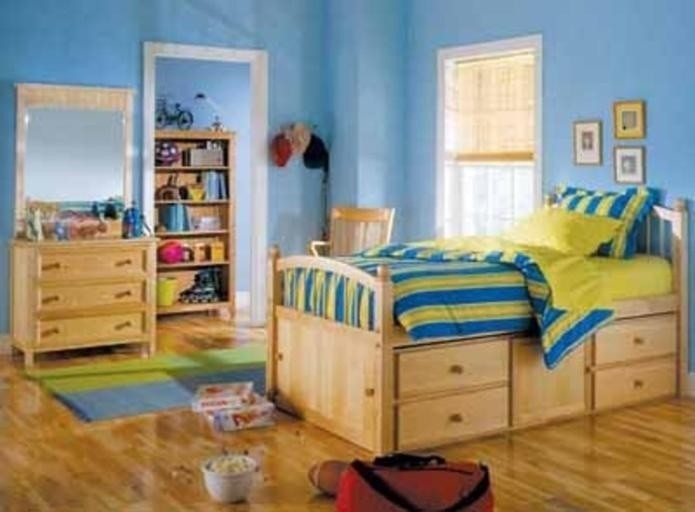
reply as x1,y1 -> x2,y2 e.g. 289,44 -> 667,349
124,202 -> 144,239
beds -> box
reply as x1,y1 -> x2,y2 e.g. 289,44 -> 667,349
264,184 -> 691,460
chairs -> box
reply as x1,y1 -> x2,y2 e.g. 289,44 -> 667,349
306,205 -> 398,261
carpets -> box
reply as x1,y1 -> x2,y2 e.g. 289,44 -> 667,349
16,344 -> 272,426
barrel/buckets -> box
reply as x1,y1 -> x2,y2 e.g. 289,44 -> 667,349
158,276 -> 176,307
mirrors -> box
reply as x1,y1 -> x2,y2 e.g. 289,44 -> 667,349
11,79 -> 134,233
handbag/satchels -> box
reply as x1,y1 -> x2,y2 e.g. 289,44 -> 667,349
337,451 -> 494,512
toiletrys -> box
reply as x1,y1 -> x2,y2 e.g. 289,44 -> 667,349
25,200 -> 45,241
193,240 -> 205,262
210,236 -> 225,261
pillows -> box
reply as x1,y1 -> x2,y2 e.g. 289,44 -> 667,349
551,186 -> 663,260
503,200 -> 625,260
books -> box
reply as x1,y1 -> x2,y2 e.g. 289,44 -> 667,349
156,204 -> 221,232
201,172 -> 226,202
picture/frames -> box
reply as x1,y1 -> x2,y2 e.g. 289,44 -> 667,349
572,119 -> 602,167
613,100 -> 646,140
613,144 -> 646,186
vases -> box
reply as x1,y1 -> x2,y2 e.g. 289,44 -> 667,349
197,457 -> 258,504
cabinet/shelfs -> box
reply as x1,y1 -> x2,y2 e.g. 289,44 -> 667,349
154,126 -> 240,323
12,238 -> 158,366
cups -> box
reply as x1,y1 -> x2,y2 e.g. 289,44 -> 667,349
157,274 -> 177,307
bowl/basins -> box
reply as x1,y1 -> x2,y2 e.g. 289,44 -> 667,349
202,452 -> 258,503
